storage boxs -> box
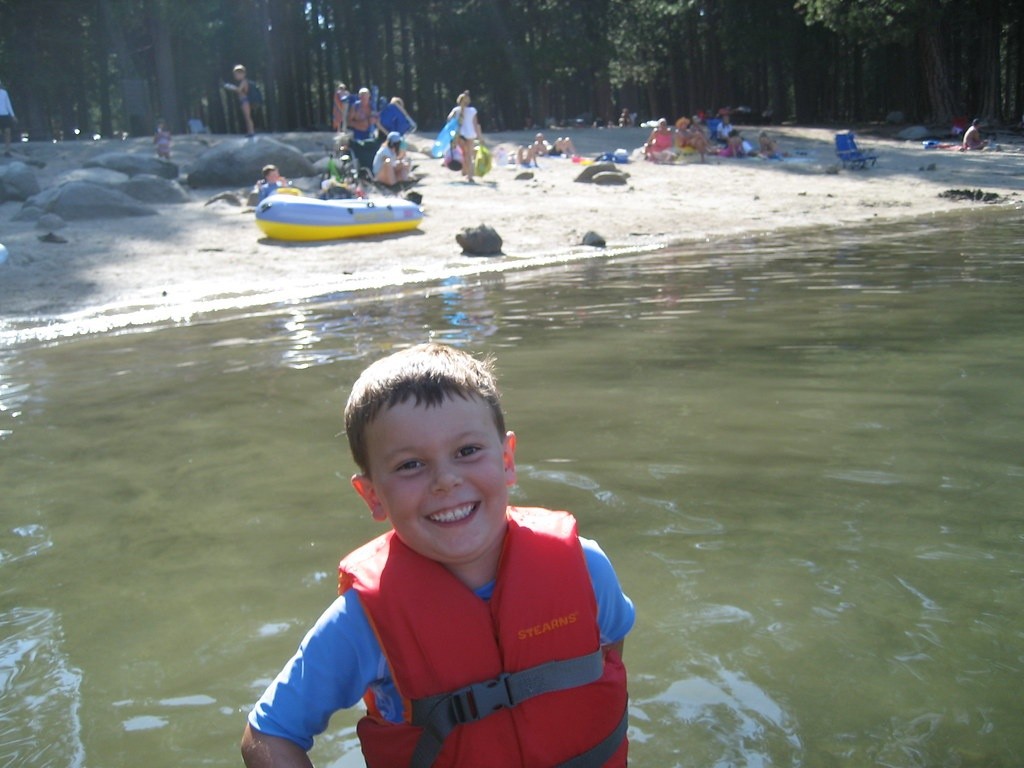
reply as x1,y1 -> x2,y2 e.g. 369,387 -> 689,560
923,140 -> 939,150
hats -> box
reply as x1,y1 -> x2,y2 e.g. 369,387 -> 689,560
387,131 -> 402,143
233,65 -> 245,73
675,117 -> 689,126
659,118 -> 667,124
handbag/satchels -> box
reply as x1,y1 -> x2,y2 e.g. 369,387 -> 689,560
475,144 -> 492,177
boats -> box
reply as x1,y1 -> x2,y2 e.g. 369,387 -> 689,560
252,192 -> 425,241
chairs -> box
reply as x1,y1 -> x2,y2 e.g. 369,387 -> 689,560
834,133 -> 878,170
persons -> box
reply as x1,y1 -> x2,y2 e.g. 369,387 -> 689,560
332,83 -> 417,187
241,342 -> 635,768
256,165 -> 286,204
153,117 -> 170,160
224,64 -> 254,137
447,93 -> 484,183
0,80 -> 18,143
962,118 -> 987,149
643,112 -> 794,163
504,133 -> 575,169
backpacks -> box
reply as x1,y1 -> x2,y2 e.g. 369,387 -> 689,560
241,79 -> 262,109
